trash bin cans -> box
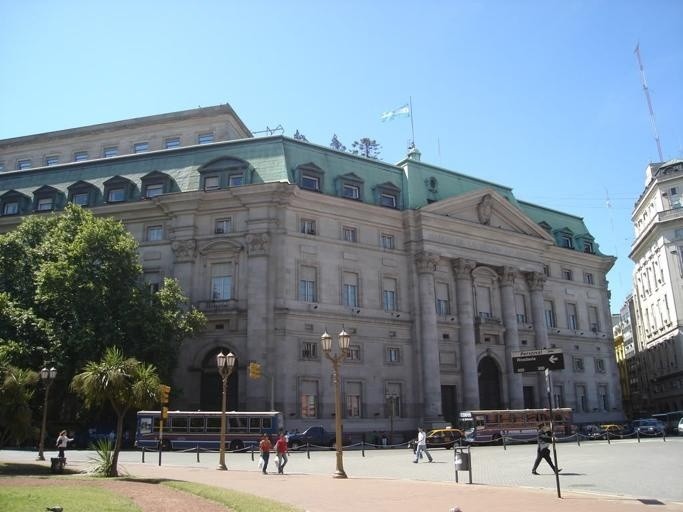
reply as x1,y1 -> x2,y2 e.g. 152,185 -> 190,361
455,446 -> 472,484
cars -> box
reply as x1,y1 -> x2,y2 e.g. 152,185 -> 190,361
581,419 -> 668,439
407,425 -> 464,451
677,417 -> 682,433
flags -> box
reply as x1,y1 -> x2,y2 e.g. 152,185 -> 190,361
380,102 -> 410,123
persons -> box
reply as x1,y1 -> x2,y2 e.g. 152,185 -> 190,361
55,429 -> 74,464
531,423 -> 563,475
274,433 -> 289,474
412,426 -> 432,463
259,432 -> 272,474
284,430 -> 289,449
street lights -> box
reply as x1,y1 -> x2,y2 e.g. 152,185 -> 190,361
214,350 -> 236,472
33,366 -> 58,461
318,326 -> 354,479
382,392 -> 399,438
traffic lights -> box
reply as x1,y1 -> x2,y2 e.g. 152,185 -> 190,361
160,385 -> 171,404
249,362 -> 257,378
253,364 -> 262,379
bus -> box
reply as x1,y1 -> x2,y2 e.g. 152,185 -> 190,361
456,407 -> 576,445
133,407 -> 283,453
650,410 -> 682,432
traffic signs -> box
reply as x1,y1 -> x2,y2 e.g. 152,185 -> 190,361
511,347 -> 566,374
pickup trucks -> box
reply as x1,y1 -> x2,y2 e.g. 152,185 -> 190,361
285,425 -> 352,449
